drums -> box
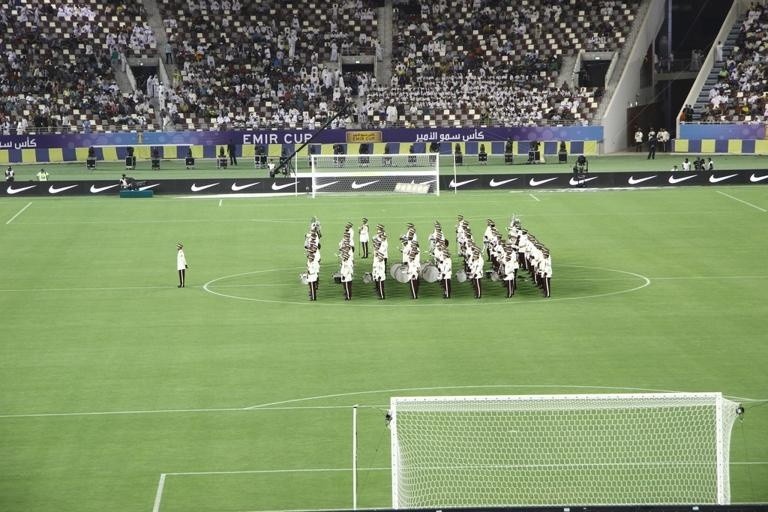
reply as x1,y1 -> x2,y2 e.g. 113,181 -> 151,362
334,273 -> 341,284
420,263 -> 439,283
362,272 -> 372,284
390,264 -> 410,283
456,270 -> 467,282
301,274 -> 309,284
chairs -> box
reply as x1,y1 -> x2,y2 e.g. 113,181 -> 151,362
0,1 -> 638,134
707,0 -> 768,124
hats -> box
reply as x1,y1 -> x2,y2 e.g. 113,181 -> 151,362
307,224 -> 317,260
514,221 -> 549,255
178,243 -> 182,248
341,221 -> 352,259
487,219 -> 511,255
435,224 -> 449,257
374,224 -> 386,259
363,218 -> 368,223
458,215 -> 481,253
406,223 -> 419,259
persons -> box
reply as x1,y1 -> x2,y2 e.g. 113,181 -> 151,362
305,214 -> 552,299
176,242 -> 188,288
